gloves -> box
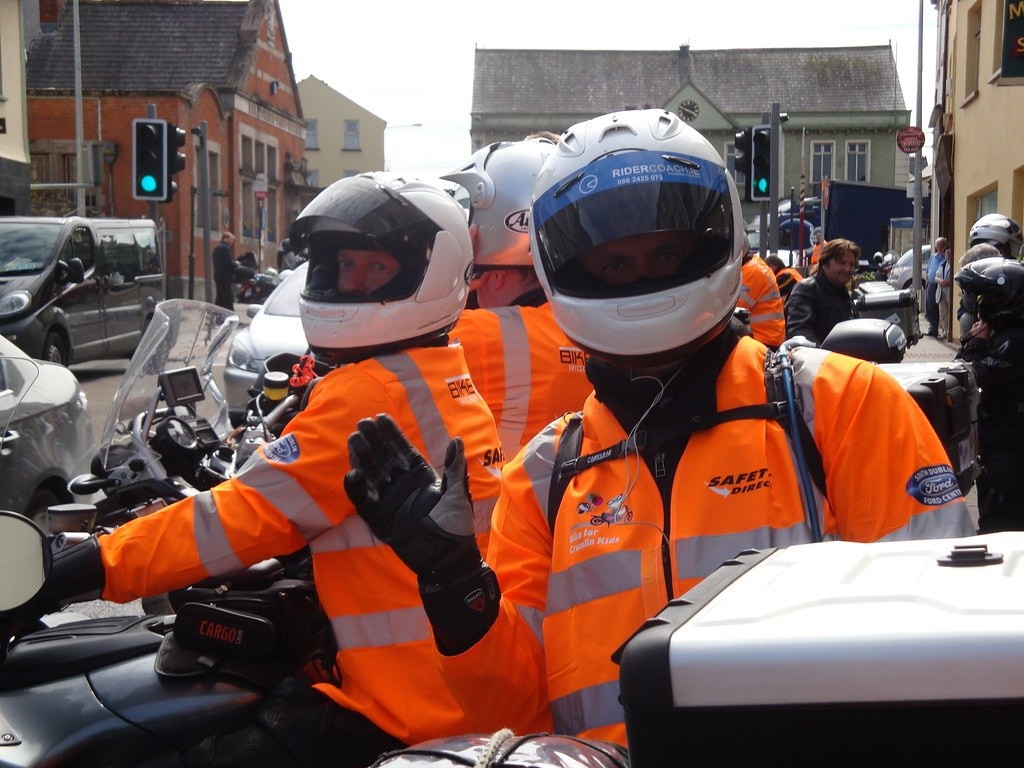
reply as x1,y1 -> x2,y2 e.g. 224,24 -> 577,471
0,536 -> 104,633
342,413 -> 501,652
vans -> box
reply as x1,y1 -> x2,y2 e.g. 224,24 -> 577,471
0,217 -> 180,376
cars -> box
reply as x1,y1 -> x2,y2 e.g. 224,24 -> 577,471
887,244 -> 936,313
0,331 -> 95,540
745,194 -> 823,259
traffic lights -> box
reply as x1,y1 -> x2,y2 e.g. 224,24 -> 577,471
159,120 -> 187,202
132,118 -> 168,201
733,125 -> 752,201
751,125 -> 771,201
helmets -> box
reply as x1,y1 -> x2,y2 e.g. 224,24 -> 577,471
967,213 -> 1022,259
975,264 -> 1024,330
953,257 -> 1007,294
441,137 -> 560,269
299,171 -> 474,362
527,108 -> 746,365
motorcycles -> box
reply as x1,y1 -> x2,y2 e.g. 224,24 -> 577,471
0,245 -> 1023,768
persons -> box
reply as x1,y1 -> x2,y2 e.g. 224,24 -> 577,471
922,237 -> 951,339
0,171 -> 507,768
212,232 -> 240,324
450,140 -> 595,464
738,227 -> 861,351
955,214 -> 1024,535
346,108 -> 976,749
